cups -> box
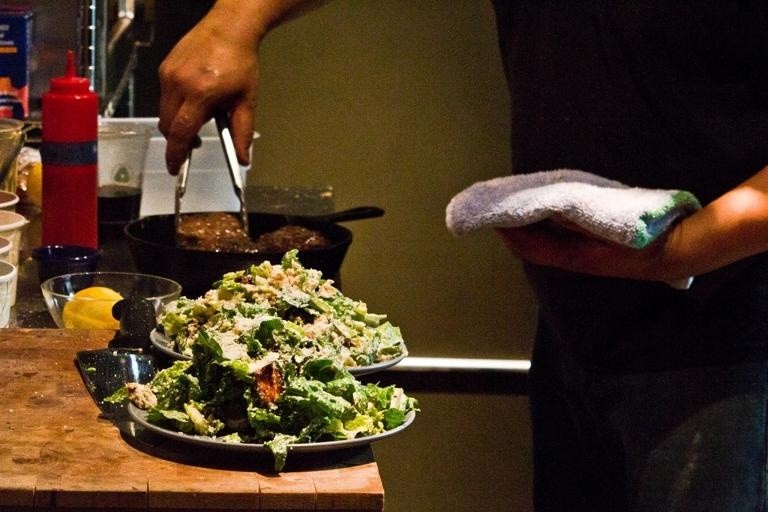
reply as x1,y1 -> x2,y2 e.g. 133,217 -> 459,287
1,115 -> 26,197
1,188 -> 34,329
98,116 -> 261,222
33,244 -> 102,288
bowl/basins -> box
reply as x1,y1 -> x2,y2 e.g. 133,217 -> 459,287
39,270 -> 181,331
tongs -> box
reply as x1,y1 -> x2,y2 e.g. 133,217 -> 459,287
173,110 -> 251,236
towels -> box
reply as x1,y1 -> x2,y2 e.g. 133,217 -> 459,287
443,167 -> 702,292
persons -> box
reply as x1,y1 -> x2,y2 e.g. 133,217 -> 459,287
107,2 -> 144,81
156,1 -> 767,512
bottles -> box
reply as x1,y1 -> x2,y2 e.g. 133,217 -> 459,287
42,49 -> 98,252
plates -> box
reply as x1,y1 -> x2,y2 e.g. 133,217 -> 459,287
127,383 -> 417,451
150,318 -> 407,379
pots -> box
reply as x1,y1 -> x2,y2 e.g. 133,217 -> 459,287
123,203 -> 387,287
